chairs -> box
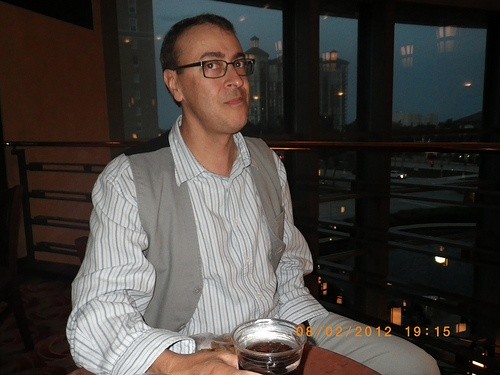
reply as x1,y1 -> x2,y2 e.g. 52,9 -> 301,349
1,184 -> 37,351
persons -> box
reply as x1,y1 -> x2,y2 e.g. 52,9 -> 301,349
66,12 -> 439,375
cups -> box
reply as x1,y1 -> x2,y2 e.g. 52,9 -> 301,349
230,318 -> 307,375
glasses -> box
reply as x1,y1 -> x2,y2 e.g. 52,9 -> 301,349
173,58 -> 255,79
